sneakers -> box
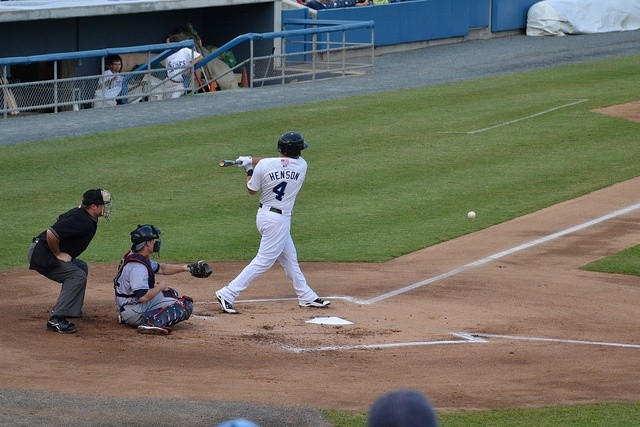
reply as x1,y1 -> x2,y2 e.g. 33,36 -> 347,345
47,319 -> 76,334
215,293 -> 236,313
299,297 -> 331,308
118,313 -> 124,324
138,323 -> 168,335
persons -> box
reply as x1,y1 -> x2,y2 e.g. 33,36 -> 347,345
306,0 -> 371,10
44,188 -> 112,334
149,35 -> 203,101
114,224 -> 213,334
214,131 -> 330,313
92,54 -> 126,108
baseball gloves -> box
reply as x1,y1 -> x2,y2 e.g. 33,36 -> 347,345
187,260 -> 212,279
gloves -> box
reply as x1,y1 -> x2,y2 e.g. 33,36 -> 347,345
236,155 -> 253,172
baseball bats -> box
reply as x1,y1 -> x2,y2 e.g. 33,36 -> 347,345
219,159 -> 242,167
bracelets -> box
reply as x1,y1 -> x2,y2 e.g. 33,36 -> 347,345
246,169 -> 254,175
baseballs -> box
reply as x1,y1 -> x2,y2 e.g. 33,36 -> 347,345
467,211 -> 476,219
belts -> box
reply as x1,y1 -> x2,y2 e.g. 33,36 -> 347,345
32,238 -> 38,243
166,75 -> 184,83
260,203 -> 281,214
120,306 -> 125,312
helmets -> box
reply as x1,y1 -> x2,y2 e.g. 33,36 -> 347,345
130,224 -> 161,259
278,131 -> 308,158
82,189 -> 114,218
